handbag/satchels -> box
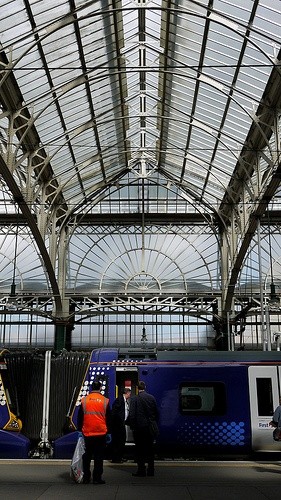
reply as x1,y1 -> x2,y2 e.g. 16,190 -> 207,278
72,438 -> 85,484
272,428 -> 280,442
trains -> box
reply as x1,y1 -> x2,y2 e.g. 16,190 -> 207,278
0,347 -> 281,461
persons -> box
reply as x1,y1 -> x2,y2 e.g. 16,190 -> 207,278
77,381 -> 112,485
110,388 -> 131,463
269,396 -> 281,429
125,381 -> 161,476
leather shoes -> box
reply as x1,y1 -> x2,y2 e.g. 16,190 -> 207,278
112,458 -> 127,463
131,471 -> 156,477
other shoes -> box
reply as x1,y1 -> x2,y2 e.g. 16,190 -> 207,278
92,479 -> 106,484
83,479 -> 91,483
